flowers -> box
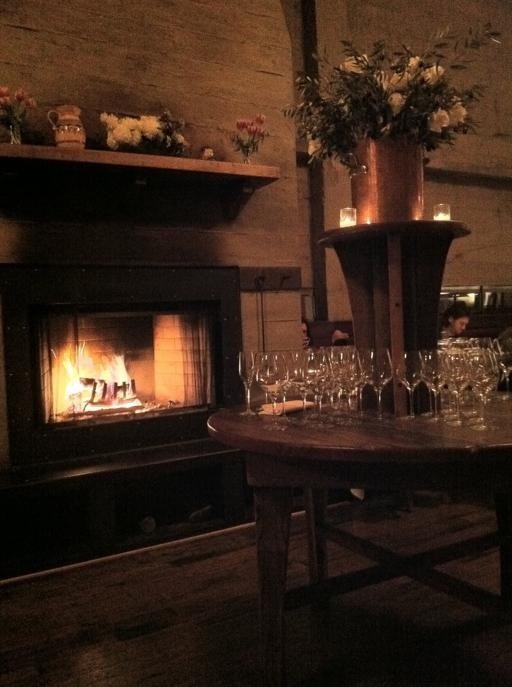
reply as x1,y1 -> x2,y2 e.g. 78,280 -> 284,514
283,25 -> 498,161
0,85 -> 37,141
99,108 -> 269,163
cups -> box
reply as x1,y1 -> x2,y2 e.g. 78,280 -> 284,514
340,207 -> 357,227
433,203 -> 451,221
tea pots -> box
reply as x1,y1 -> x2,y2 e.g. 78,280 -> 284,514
49,101 -> 85,150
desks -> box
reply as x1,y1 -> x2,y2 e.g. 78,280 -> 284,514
203,390 -> 512,685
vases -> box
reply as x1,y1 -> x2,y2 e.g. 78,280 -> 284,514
9,116 -> 21,144
346,131 -> 424,224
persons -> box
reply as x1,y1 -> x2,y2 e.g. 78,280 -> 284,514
436,303 -> 470,340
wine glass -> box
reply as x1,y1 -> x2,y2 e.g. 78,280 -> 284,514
237,337 -> 507,430
